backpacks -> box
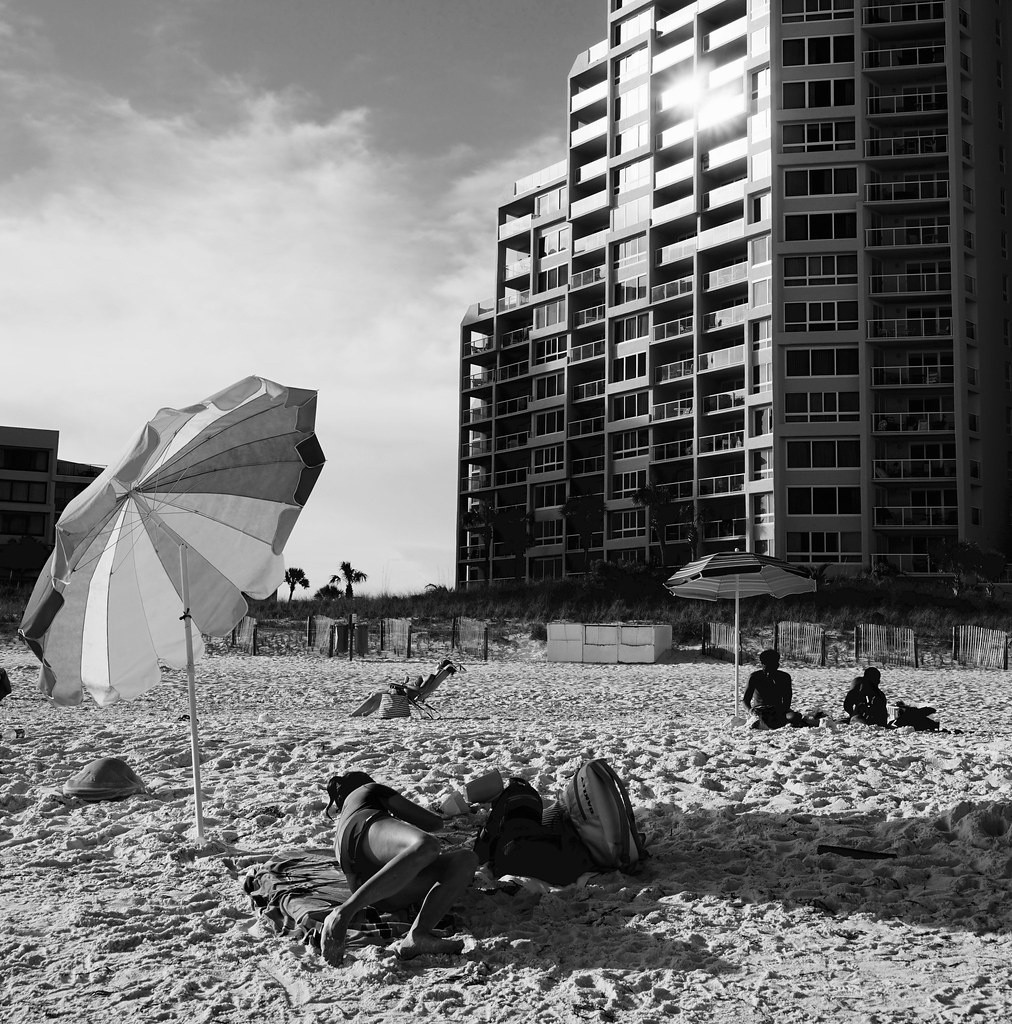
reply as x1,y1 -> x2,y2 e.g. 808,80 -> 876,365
564,755 -> 647,871
485,776 -> 544,878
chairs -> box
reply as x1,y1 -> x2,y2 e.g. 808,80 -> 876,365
349,663 -> 456,721
904,327 -> 916,336
878,326 -> 891,336
906,140 -> 916,153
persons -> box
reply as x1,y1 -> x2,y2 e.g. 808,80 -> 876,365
322,772 -> 479,966
350,660 -> 453,716
844,666 -> 890,725
743,650 -> 802,729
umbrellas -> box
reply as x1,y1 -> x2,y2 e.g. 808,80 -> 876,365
18,376 -> 324,836
661,549 -> 818,715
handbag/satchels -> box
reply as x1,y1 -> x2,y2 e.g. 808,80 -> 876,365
378,691 -> 410,718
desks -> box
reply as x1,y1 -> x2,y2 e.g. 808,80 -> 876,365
914,102 -> 939,110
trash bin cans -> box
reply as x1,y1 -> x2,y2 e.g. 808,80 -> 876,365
334,623 -> 349,655
354,623 -> 369,656
